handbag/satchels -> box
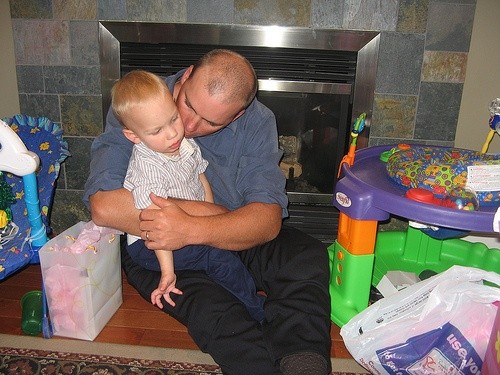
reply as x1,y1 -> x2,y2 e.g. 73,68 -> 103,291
339,264 -> 500,375
38,220 -> 123,341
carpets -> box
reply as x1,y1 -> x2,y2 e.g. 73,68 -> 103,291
0,329 -> 375,374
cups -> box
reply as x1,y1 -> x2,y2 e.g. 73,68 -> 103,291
21,291 -> 49,336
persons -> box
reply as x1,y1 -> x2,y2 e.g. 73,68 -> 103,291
109,69 -> 266,327
82,48 -> 332,375
